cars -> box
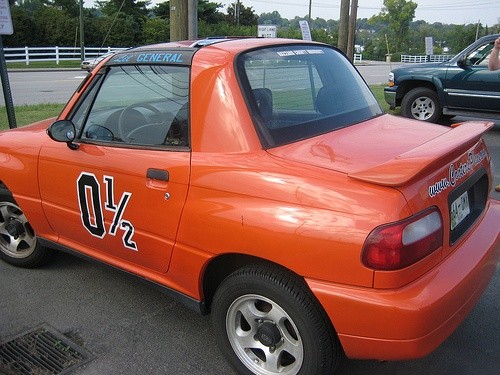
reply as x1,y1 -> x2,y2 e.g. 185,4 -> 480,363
1,35 -> 500,375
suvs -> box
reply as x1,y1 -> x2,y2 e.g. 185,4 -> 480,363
383,33 -> 500,124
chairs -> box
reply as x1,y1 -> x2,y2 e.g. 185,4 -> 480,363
315,84 -> 347,115
162,102 -> 189,146
251,88 -> 274,128
